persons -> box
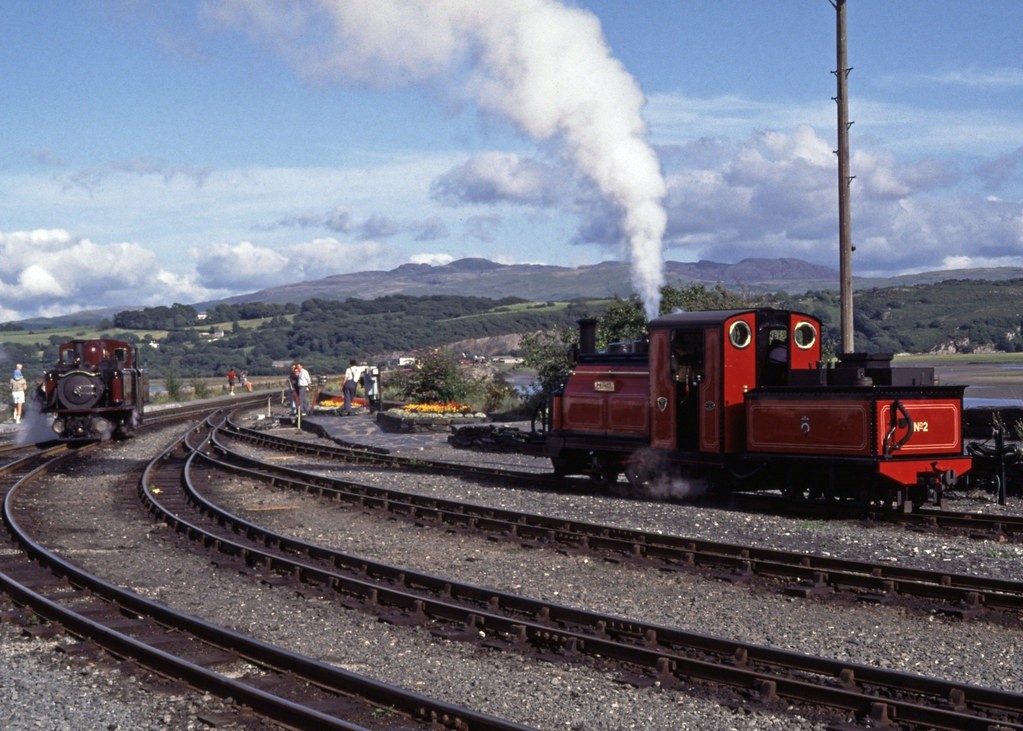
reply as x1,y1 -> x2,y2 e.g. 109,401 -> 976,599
239,371 -> 252,392
226,369 -> 237,396
10,377 -> 28,424
12,363 -> 24,381
340,359 -> 370,415
289,362 -> 312,416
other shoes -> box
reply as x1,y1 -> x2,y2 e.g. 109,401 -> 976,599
16,417 -> 22,424
347,412 -> 352,416
13,412 -> 17,420
337,408 -> 343,417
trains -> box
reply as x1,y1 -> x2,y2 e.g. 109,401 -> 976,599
29,337 -> 150,451
530,306 -> 974,525
